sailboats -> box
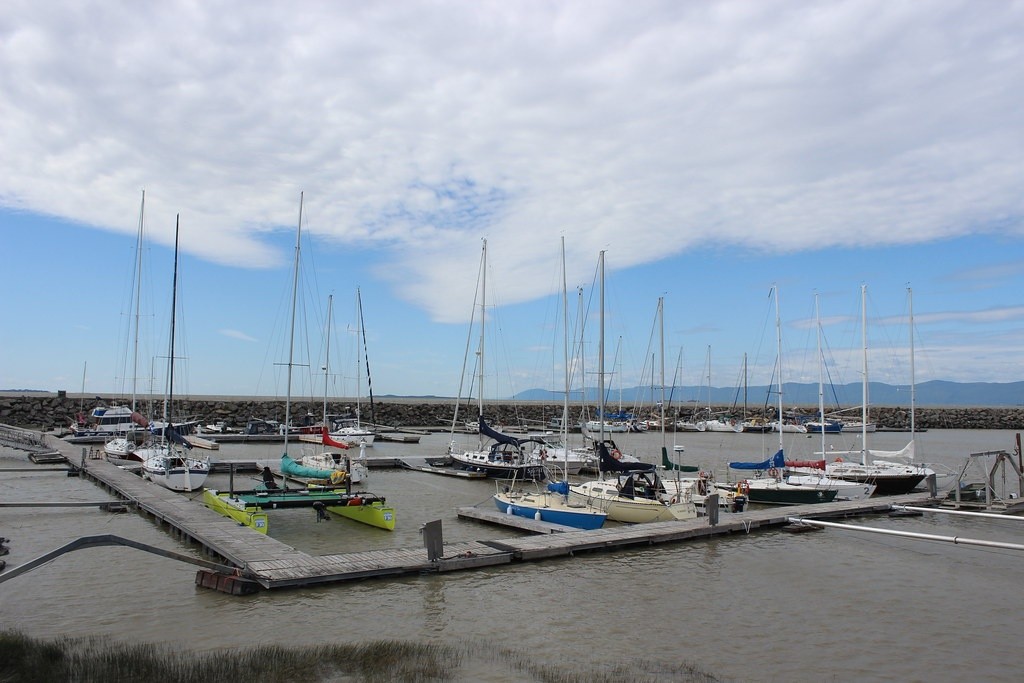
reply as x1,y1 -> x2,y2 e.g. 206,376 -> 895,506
559,251 -> 701,525
140,212 -> 213,493
526,285 -> 660,477
705,283 -> 839,507
100,193 -> 187,469
70,188 -> 207,439
277,190 -> 347,487
825,282 -> 937,494
580,282 -> 878,436
487,237 -> 612,533
326,289 -> 377,445
779,293 -> 879,501
422,238 -> 557,485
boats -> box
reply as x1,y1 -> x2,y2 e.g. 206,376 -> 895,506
653,413 -> 750,513
945,480 -> 993,502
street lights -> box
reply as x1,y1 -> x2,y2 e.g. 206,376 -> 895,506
297,294 -> 370,485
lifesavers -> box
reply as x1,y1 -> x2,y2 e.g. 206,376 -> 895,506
611,450 -> 622,460
738,480 -> 749,494
768,468 -> 778,477
835,457 -> 843,462
126,443 -> 136,453
